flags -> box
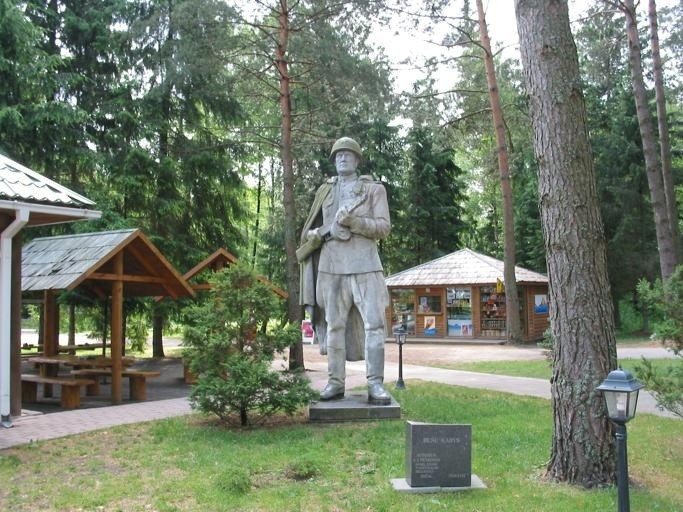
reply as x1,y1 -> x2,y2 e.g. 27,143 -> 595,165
495,278 -> 505,294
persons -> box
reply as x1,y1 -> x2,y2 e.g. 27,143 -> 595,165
297,135 -> 395,405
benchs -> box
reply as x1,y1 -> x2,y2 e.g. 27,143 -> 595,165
16,347 -> 160,410
